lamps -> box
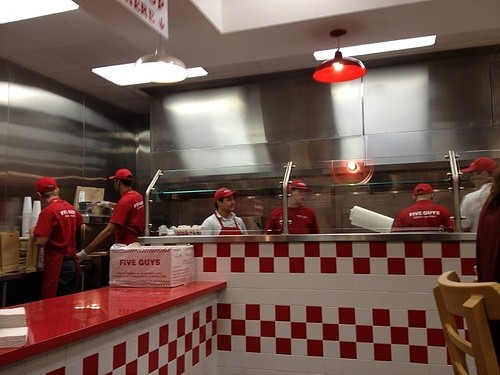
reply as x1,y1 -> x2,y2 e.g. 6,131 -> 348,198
313,28 -> 367,83
329,161 -> 375,185
135,33 -> 187,84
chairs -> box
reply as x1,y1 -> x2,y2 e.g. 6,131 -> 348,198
433,270 -> 500,375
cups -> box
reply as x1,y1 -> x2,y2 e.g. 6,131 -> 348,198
21,196 -> 42,237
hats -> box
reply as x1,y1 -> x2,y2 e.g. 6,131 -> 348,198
289,180 -> 311,191
34,176 -> 58,192
462,157 -> 496,173
414,183 -> 433,195
213,187 -> 236,201
109,168 -> 134,181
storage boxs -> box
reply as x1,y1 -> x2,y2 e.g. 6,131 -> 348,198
110,245 -> 194,288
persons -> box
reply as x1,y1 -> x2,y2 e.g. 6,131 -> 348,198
198,187 -> 247,235
393,183 -> 455,232
73,168 -> 145,260
460,157 -> 496,232
29,177 -> 84,300
262,179 -> 319,234
475,167 -> 500,363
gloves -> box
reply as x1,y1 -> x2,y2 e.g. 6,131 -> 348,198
76,249 -> 87,262
97,200 -> 110,209
